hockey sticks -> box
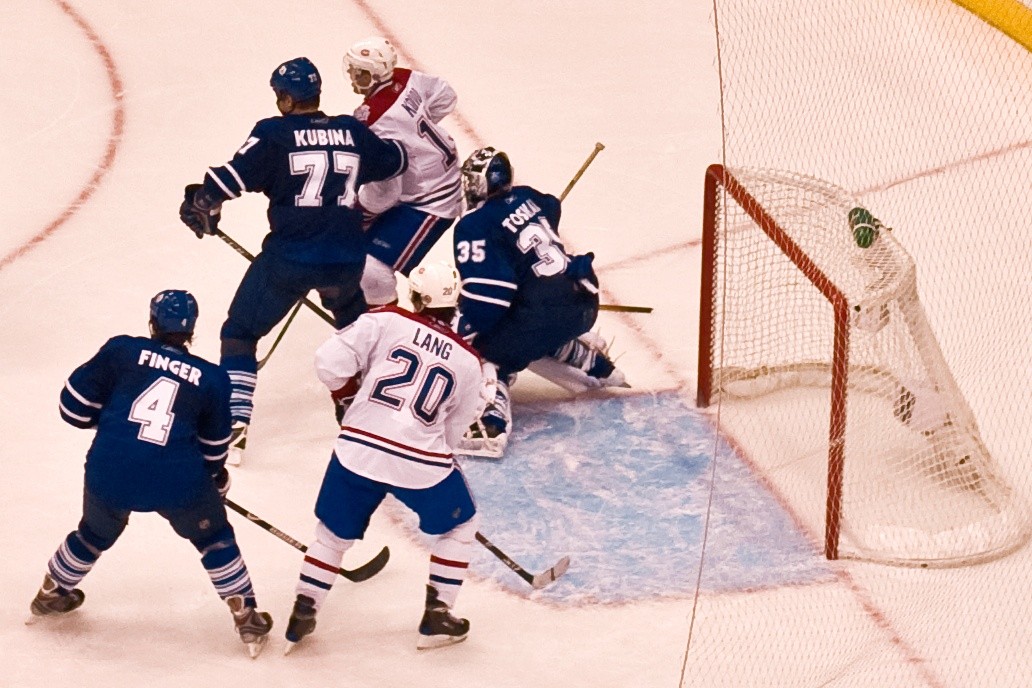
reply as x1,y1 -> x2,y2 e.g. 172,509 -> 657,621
557,140 -> 607,201
224,497 -> 390,584
473,530 -> 570,589
596,303 -> 653,314
256,287 -> 312,371
215,227 -> 337,328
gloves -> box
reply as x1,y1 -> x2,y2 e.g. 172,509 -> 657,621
180,183 -> 221,239
333,376 -> 363,409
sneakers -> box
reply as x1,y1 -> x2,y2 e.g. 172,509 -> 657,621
584,352 -> 630,389
225,594 -> 272,658
415,583 -> 470,649
283,594 -> 317,655
25,573 -> 85,626
225,427 -> 246,464
453,416 -> 506,458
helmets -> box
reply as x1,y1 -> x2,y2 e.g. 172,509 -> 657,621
149,290 -> 199,333
271,57 -> 322,103
459,146 -> 514,216
347,38 -> 398,96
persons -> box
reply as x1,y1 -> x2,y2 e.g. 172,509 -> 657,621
284,257 -> 486,643
31,291 -> 273,645
179,38 -> 625,450
